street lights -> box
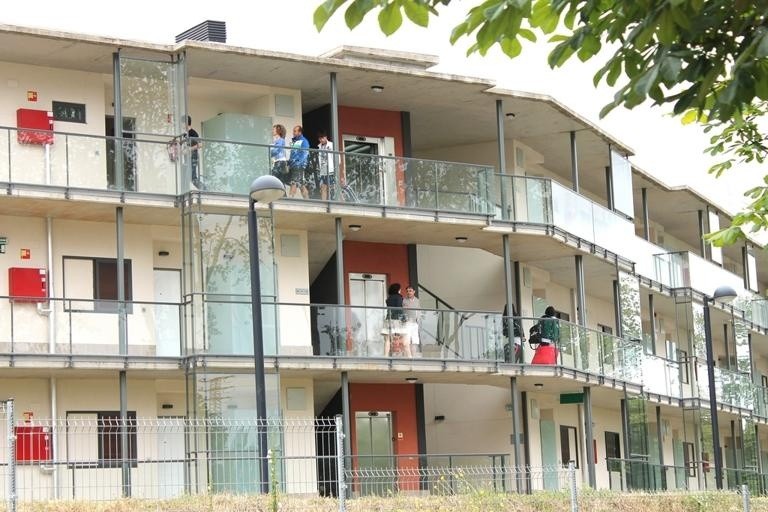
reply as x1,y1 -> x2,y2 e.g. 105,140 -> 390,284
703,285 -> 738,490
247,174 -> 286,494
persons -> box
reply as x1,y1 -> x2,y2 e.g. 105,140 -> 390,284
501,303 -> 525,363
316,131 -> 345,203
400,284 -> 421,357
538,305 -> 560,346
179,114 -> 207,190
270,124 -> 289,197
382,282 -> 413,358
287,126 -> 308,199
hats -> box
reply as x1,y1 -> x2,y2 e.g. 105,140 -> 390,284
545,305 -> 556,318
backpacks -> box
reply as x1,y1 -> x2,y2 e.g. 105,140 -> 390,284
529,318 -> 546,344
